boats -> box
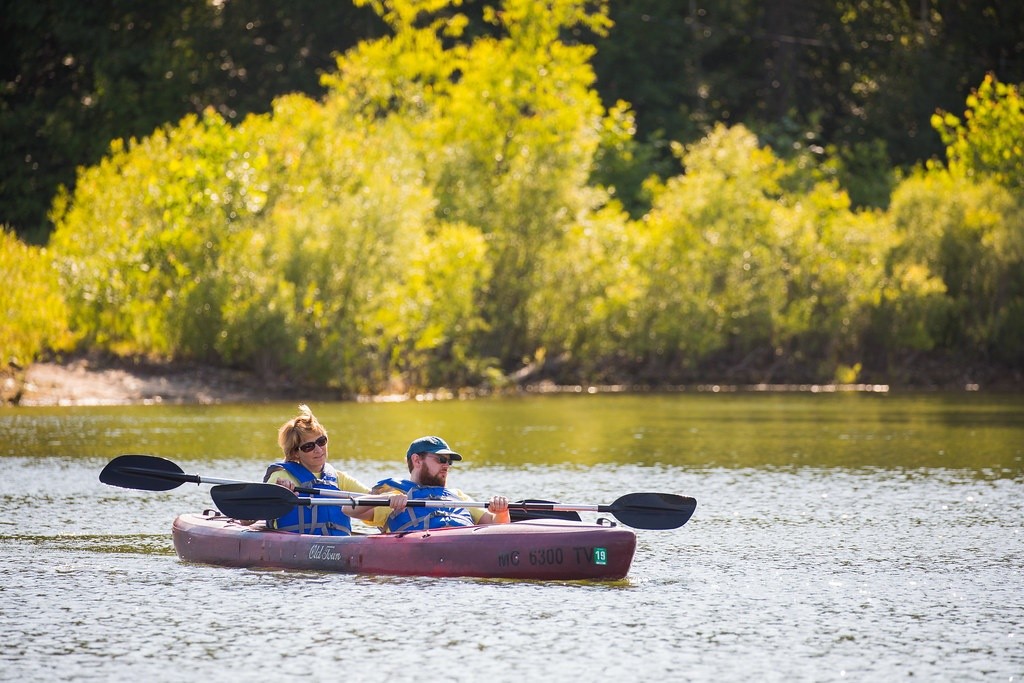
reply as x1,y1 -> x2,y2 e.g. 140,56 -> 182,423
171,506 -> 638,589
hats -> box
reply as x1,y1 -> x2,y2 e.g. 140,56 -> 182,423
407,436 -> 462,461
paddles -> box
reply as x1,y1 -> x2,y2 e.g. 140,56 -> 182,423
208,482 -> 699,531
98,454 -> 374,498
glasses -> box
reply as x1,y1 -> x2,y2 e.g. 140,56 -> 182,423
295,436 -> 327,453
423,456 -> 453,465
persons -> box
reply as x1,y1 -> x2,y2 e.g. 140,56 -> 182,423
239,404 -> 371,536
339,436 -> 510,533
